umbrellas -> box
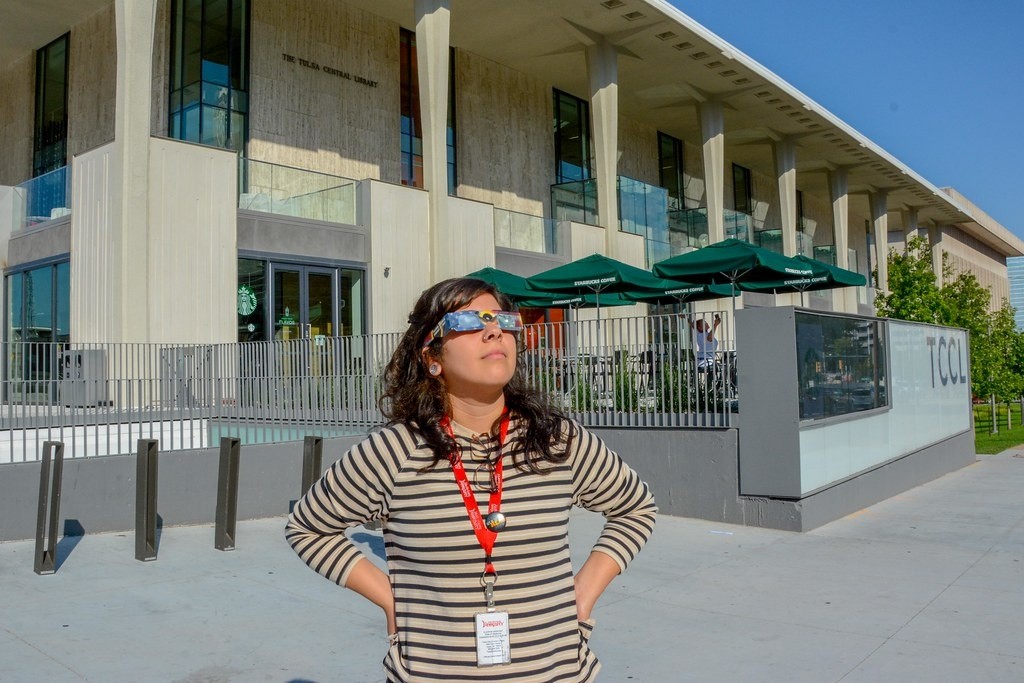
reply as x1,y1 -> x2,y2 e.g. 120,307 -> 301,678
466,238 -> 865,357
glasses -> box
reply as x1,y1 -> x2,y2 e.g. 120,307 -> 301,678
470,432 -> 498,493
422,309 -> 524,346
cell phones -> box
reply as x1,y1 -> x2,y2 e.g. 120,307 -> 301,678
715,314 -> 720,322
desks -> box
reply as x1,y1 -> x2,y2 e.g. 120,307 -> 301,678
696,350 -> 737,402
563,355 -> 636,399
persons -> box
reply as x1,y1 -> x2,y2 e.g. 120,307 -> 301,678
284,279 -> 659,683
679,312 -> 721,371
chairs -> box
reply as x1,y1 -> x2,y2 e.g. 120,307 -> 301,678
528,350 -> 737,404
